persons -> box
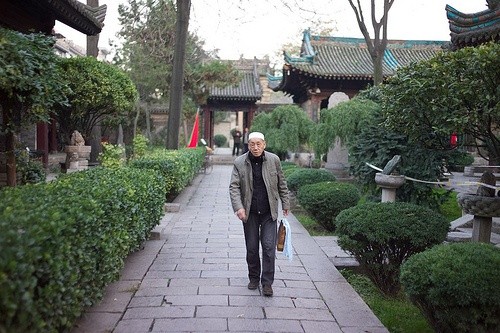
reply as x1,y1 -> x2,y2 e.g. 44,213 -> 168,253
242,128 -> 250,154
229,132 -> 290,298
231,127 -> 242,156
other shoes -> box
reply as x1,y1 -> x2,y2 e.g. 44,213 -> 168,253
248,281 -> 259,289
262,284 -> 273,295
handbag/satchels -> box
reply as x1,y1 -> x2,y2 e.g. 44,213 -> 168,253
277,218 -> 292,259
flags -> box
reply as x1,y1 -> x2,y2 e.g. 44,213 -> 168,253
187,107 -> 199,147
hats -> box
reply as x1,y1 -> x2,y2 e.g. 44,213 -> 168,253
249,132 -> 265,141
